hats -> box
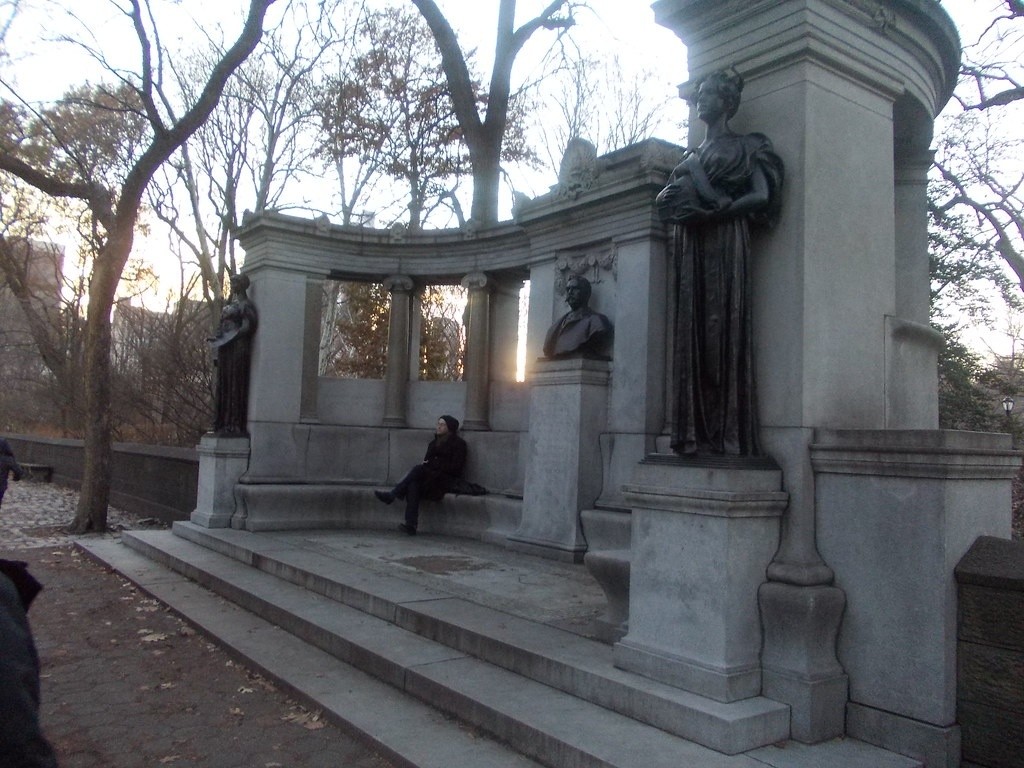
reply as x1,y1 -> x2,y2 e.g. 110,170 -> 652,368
440,414 -> 459,432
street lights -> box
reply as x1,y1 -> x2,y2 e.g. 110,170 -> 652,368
1002,395 -> 1015,418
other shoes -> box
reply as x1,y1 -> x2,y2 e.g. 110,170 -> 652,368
375,490 -> 395,504
400,523 -> 416,535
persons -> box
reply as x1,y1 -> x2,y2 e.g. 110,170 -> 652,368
374,414 -> 469,535
655,69 -> 785,458
206,273 -> 258,434
543,274 -> 610,359
0,437 -> 22,507
